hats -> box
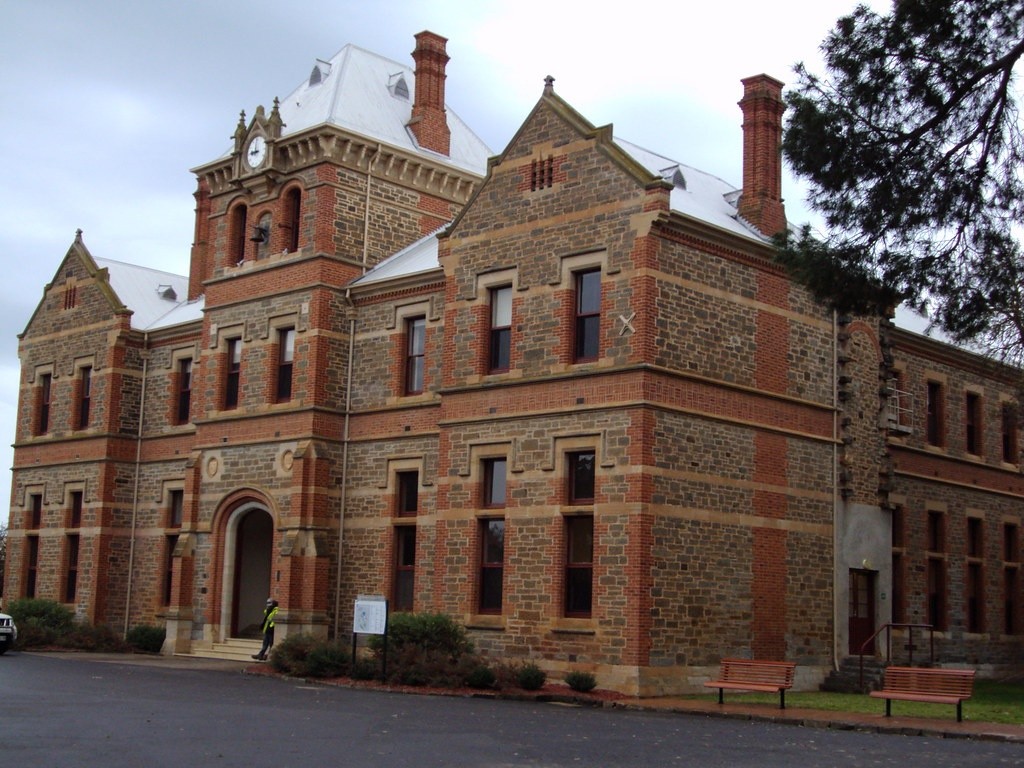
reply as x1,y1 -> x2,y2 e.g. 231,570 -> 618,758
266,598 -> 275,607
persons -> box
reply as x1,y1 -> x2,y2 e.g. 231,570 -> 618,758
252,598 -> 278,661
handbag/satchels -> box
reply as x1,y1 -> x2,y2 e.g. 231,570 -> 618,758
260,623 -> 264,630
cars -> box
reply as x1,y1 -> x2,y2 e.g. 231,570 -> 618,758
0,612 -> 18,655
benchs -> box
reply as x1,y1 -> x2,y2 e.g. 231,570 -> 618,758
870,665 -> 976,722
704,656 -> 797,708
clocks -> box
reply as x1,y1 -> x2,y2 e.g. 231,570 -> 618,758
246,135 -> 265,167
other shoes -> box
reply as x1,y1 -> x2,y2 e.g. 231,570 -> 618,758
251,651 -> 268,660
